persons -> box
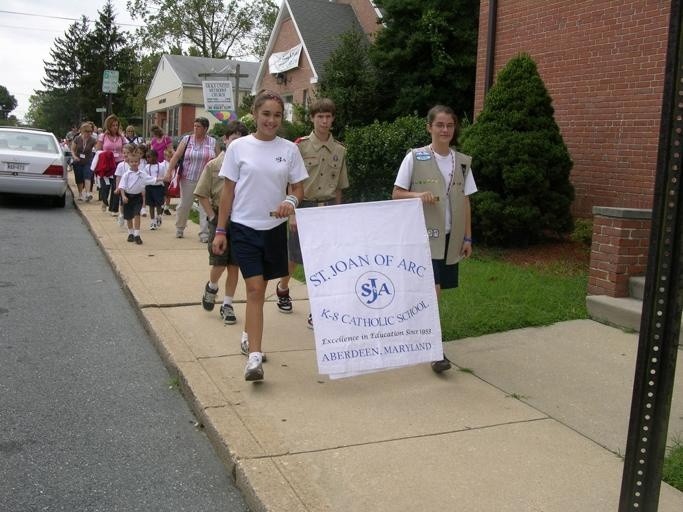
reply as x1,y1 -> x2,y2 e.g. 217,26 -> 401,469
194,121 -> 249,325
275,97 -> 349,327
114,144 -> 180,244
119,124 -> 146,144
213,88 -> 309,382
149,125 -> 173,161
392,102 -> 481,371
164,117 -> 221,243
58,121 -> 104,202
96,115 -> 127,215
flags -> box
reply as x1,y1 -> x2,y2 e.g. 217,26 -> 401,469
294,199 -> 444,380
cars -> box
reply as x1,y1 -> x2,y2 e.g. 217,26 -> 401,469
0,126 -> 72,208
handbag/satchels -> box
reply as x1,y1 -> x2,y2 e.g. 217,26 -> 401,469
167,174 -> 181,197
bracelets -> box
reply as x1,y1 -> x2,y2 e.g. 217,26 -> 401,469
285,195 -> 299,207
464,235 -> 472,245
214,227 -> 225,234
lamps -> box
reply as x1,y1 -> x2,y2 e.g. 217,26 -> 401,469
275,72 -> 287,87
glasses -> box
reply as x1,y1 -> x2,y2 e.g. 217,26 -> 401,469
431,122 -> 455,129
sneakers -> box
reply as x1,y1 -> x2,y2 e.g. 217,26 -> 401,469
202,281 -> 218,312
77,191 -> 210,244
275,281 -> 294,313
431,359 -> 451,374
241,331 -> 266,361
243,352 -> 264,381
220,304 -> 236,324
